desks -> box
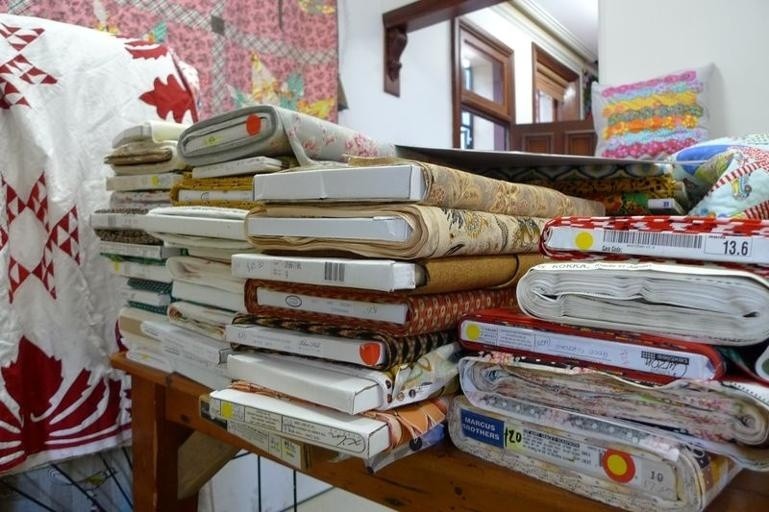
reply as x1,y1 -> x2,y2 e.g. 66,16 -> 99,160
111,352 -> 769,511
